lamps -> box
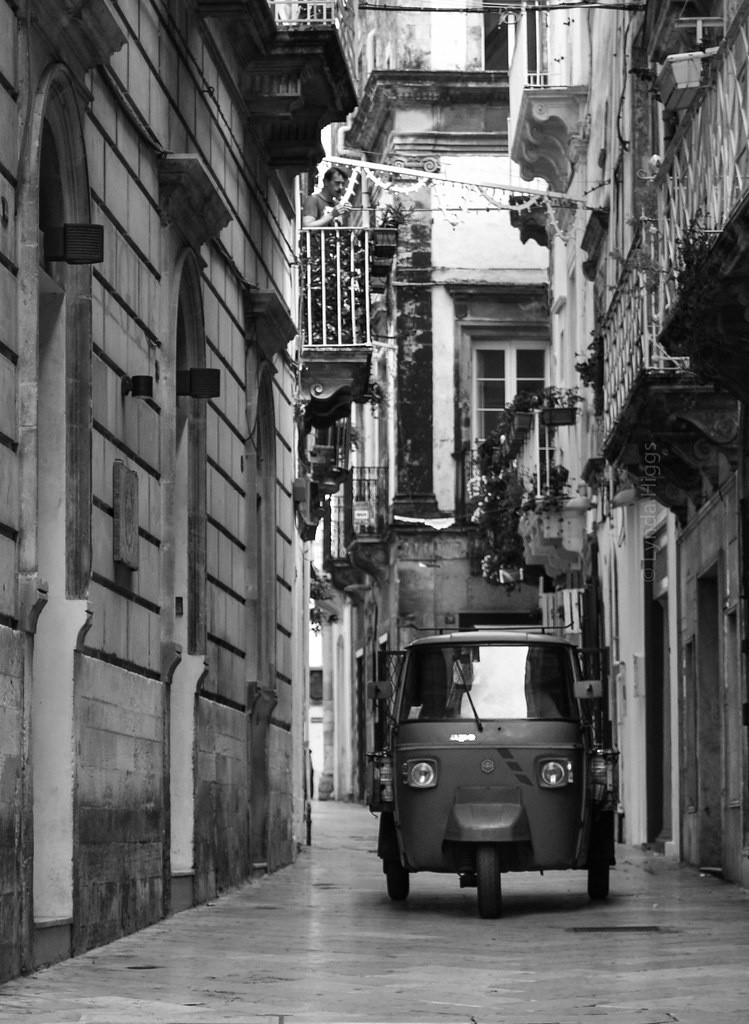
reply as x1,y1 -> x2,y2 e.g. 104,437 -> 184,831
177,367 -> 220,398
121,375 -> 152,398
43,222 -> 104,264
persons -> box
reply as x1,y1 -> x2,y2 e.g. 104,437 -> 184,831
301,166 -> 354,233
460,660 -> 559,720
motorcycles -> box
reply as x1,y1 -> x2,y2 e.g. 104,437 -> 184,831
365,627 -> 622,920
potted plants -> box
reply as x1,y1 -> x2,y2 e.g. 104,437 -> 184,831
540,384 -> 586,426
627,30 -> 724,112
490,391 -> 533,460
370,203 -> 416,293
